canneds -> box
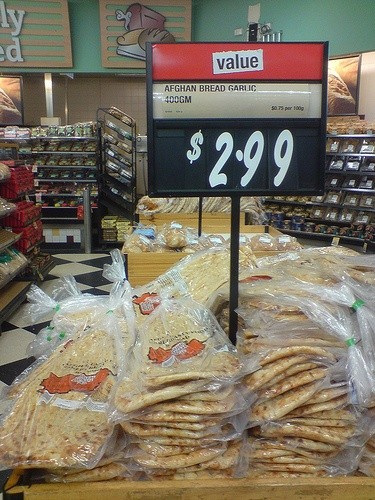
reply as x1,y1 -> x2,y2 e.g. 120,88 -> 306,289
48,121 -> 96,137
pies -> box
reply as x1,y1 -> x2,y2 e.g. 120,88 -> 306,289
0,246 -> 375,481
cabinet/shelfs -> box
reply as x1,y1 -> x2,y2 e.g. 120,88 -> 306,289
0,105 -> 375,319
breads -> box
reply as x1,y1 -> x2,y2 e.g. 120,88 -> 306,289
121,224 -> 300,254
